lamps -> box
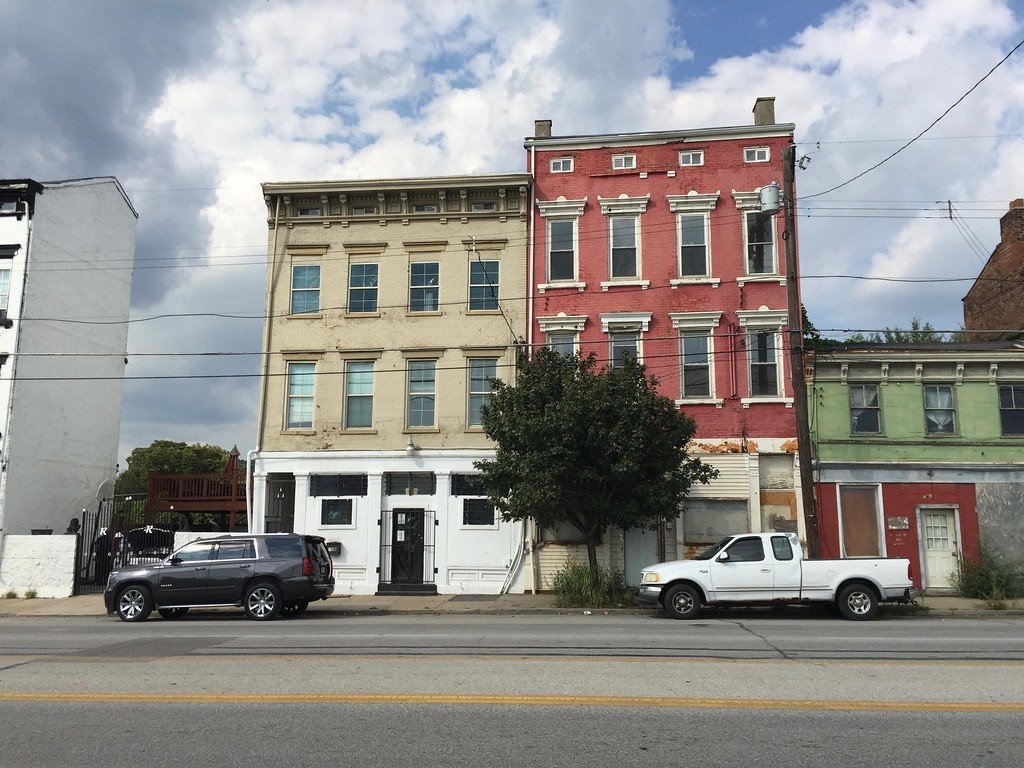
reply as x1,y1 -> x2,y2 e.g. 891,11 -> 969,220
406,435 -> 422,458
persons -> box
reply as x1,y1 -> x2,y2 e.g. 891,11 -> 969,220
94,527 -> 131,586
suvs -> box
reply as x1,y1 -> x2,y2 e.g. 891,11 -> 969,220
101,532 -> 336,623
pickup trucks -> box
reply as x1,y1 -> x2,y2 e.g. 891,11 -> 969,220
634,530 -> 920,622
